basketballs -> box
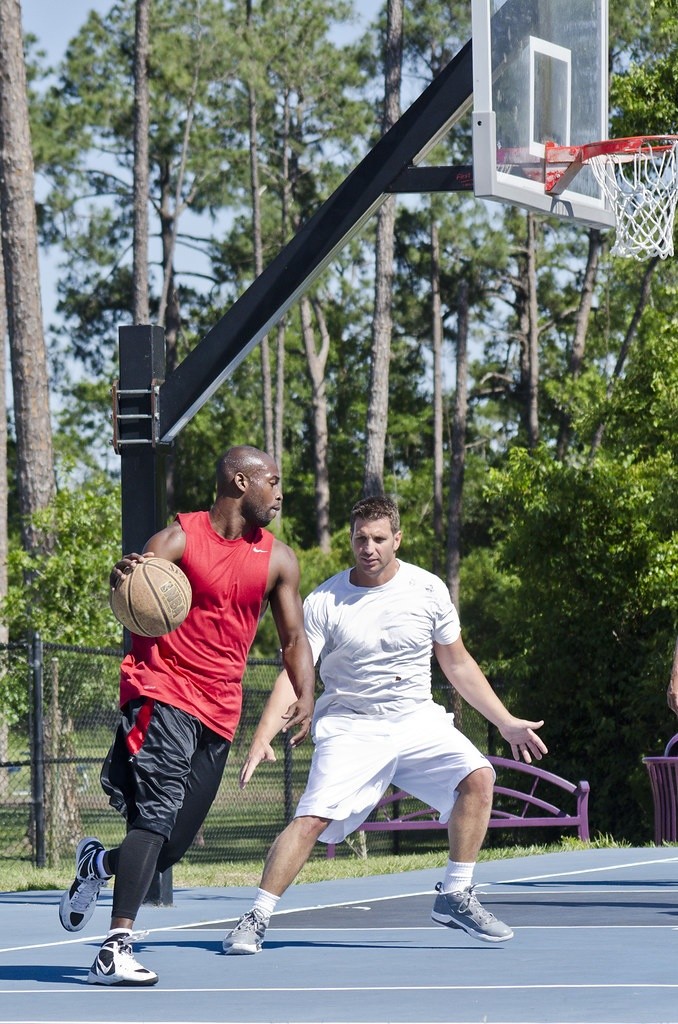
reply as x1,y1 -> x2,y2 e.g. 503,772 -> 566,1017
108,557 -> 194,639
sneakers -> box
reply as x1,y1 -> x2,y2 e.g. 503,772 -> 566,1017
59,837 -> 110,932
87,933 -> 159,986
431,881 -> 514,942
222,909 -> 269,955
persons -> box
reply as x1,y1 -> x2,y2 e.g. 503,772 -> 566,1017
221,497 -> 548,957
666,634 -> 678,718
60,442 -> 316,985
